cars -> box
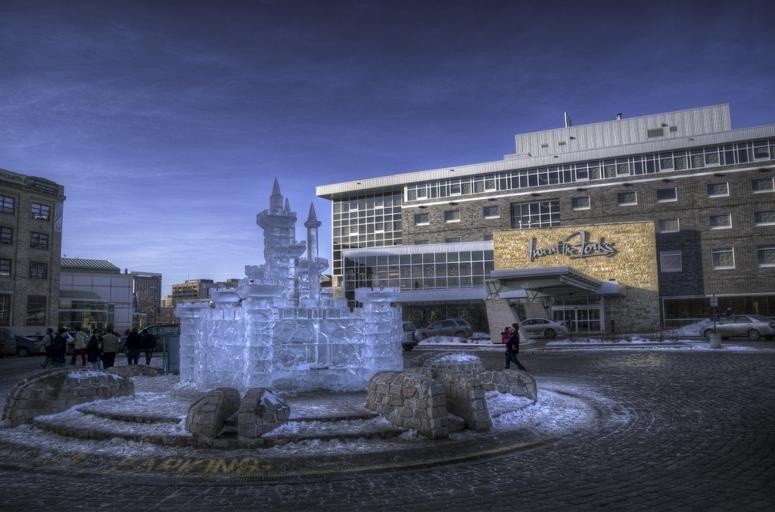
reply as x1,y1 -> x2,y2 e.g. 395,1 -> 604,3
699,313 -> 775,343
1,322 -> 181,354
423,317 -> 473,339
512,316 -> 568,340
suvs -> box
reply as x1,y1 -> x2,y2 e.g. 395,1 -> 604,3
401,320 -> 423,350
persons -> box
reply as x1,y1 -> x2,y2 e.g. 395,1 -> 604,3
35,326 -> 156,369
501,323 -> 527,372
